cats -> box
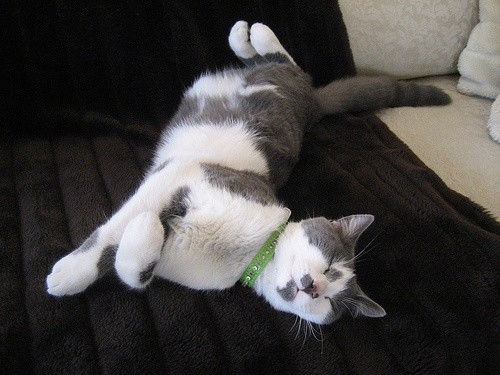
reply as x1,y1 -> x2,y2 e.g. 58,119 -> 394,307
46,19 -> 452,355
